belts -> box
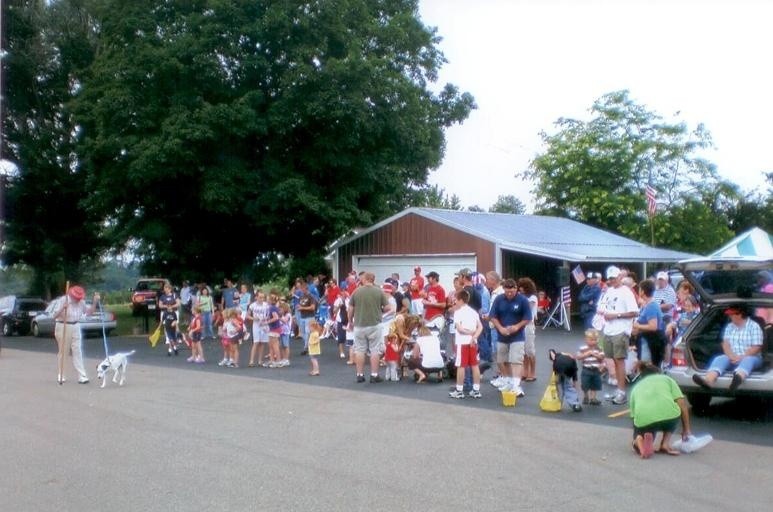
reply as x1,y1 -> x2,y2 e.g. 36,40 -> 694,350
56,320 -> 78,325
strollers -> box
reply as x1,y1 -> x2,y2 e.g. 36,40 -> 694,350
316,304 -> 338,342
397,313 -> 447,380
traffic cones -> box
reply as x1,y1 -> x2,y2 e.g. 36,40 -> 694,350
538,370 -> 561,412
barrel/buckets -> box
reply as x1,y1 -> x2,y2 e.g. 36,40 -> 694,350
501,390 -> 518,407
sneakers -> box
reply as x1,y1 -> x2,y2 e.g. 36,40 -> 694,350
57,374 -> 66,381
692,374 -> 712,391
187,356 -> 205,363
449,386 -> 482,398
573,391 -> 627,412
357,375 -> 365,382
262,354 -> 290,368
78,376 -> 89,383
490,375 -> 537,397
218,360 -> 239,368
730,375 -> 741,392
370,374 -> 384,383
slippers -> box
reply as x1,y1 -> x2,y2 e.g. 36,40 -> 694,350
642,433 -> 652,459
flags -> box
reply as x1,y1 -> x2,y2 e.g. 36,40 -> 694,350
645,165 -> 657,218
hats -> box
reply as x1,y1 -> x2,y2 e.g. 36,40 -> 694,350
724,306 -> 748,315
69,285 -> 84,301
454,269 -> 472,277
425,271 -> 438,280
607,267 -> 620,279
657,271 -> 669,281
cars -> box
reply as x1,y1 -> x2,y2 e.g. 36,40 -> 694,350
658,255 -> 772,417
126,276 -> 179,316
0,294 -> 51,339
30,296 -> 118,339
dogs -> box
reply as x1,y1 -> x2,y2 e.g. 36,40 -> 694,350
96,348 -> 137,388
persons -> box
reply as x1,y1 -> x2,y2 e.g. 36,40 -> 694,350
159,276 -> 349,375
550,263 -> 700,409
693,308 -> 764,391
626,360 -> 694,460
48,284 -> 102,385
348,265 -> 548,397
